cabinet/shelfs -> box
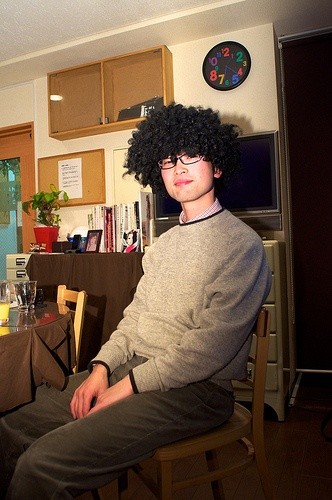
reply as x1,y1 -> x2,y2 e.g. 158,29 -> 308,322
145,238 -> 289,421
48,44 -> 172,142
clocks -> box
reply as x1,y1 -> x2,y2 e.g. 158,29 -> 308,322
201,42 -> 252,93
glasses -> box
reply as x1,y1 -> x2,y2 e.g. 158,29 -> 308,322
161,152 -> 202,169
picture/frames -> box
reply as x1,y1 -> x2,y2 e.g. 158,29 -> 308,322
85,230 -> 103,253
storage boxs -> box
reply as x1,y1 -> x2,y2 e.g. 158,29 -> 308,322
51,242 -> 72,253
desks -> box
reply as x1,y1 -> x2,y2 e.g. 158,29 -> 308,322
0,252 -> 147,416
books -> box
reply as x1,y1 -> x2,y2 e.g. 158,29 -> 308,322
86,190 -> 155,253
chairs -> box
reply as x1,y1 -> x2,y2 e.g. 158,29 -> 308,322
55,283 -> 277,500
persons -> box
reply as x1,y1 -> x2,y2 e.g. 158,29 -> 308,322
0,103 -> 272,500
87,233 -> 97,251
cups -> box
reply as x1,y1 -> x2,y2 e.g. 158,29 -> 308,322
0,325 -> 10,336
0,280 -> 11,325
17,310 -> 37,331
14,280 -> 37,310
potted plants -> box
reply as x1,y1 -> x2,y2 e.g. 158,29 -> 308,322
20,184 -> 70,253
